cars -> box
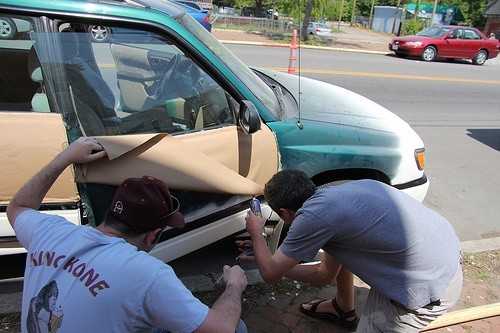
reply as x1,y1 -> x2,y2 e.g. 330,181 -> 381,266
306,21 -> 333,37
165,0 -> 212,34
0,0 -> 431,281
389,25 -> 500,65
241,6 -> 279,21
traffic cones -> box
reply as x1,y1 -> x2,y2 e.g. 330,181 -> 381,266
288,29 -> 297,74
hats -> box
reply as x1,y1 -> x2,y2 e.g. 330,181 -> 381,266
109,175 -> 185,228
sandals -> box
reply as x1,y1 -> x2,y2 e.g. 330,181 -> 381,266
300,297 -> 359,328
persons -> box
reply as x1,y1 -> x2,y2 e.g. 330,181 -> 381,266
6,136 -> 247,333
236,169 -> 464,333
